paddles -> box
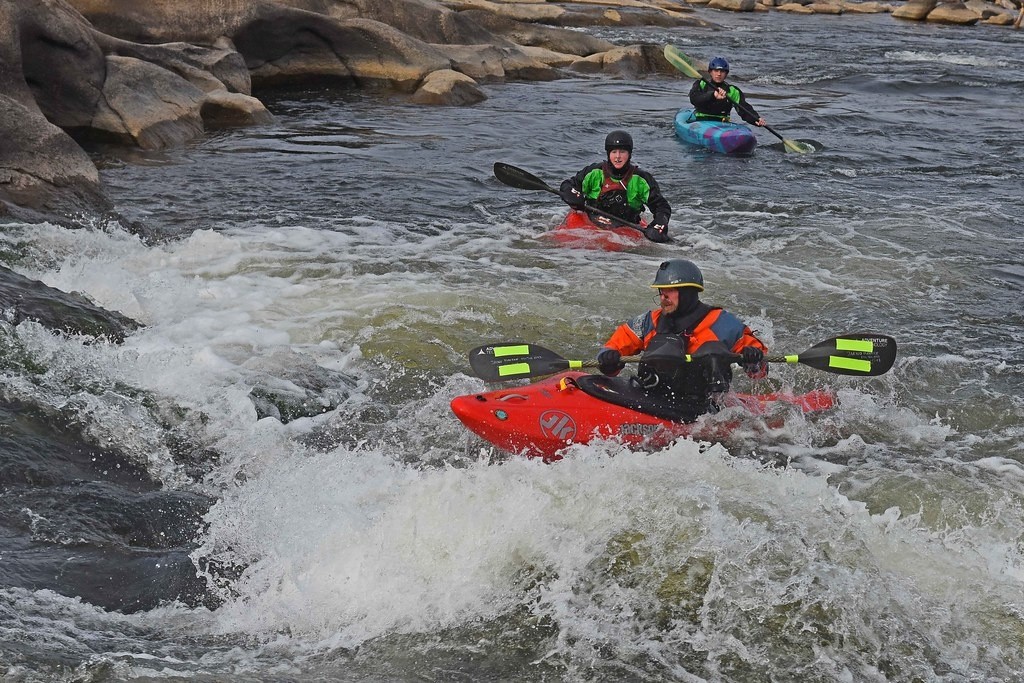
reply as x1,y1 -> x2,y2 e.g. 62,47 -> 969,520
468,333 -> 897,383
663,45 -> 811,153
493,162 -> 691,247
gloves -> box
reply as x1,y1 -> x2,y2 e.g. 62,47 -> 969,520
645,220 -> 669,243
599,351 -> 626,375
563,188 -> 584,209
741,346 -> 762,374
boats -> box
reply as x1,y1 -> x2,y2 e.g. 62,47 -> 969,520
673,107 -> 758,154
449,370 -> 834,461
548,210 -> 644,251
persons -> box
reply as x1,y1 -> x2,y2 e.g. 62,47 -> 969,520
598,259 -> 769,412
560,130 -> 672,242
690,58 -> 766,127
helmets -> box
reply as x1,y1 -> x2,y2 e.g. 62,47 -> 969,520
650,260 -> 706,290
605,132 -> 633,153
708,59 -> 729,75
